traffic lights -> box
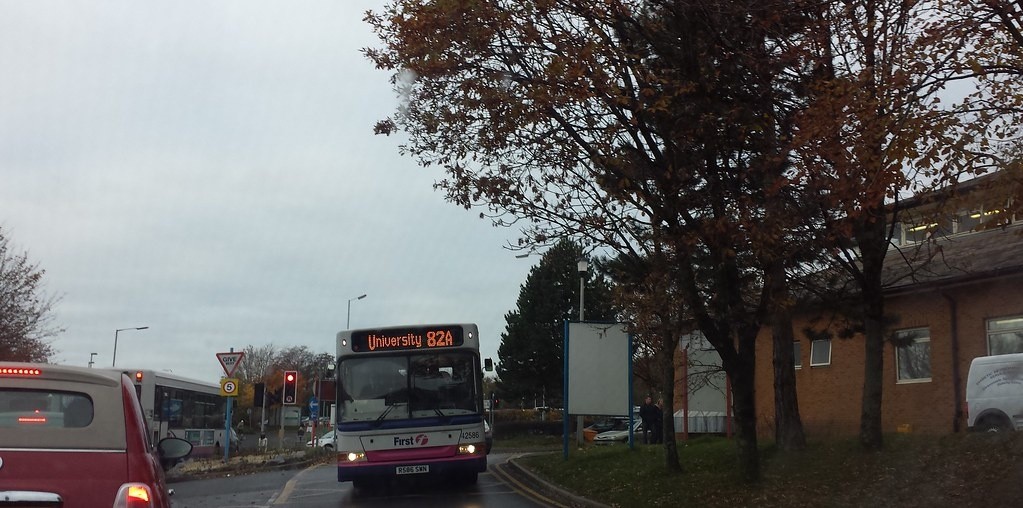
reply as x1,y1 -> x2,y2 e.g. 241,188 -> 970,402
283,371 -> 297,406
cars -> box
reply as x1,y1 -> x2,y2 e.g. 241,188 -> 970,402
592,417 -> 652,447
581,417 -> 636,445
0,361 -> 192,507
309,430 -> 336,454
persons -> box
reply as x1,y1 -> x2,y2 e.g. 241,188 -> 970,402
297,426 -> 304,442
651,399 -> 663,444
639,396 -> 654,446
237,419 -> 245,441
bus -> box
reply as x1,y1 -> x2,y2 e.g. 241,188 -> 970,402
336,320 -> 489,496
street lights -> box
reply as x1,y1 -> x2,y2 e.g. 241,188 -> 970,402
346,293 -> 367,330
90,352 -> 98,367
111,326 -> 150,366
575,256 -> 590,451
